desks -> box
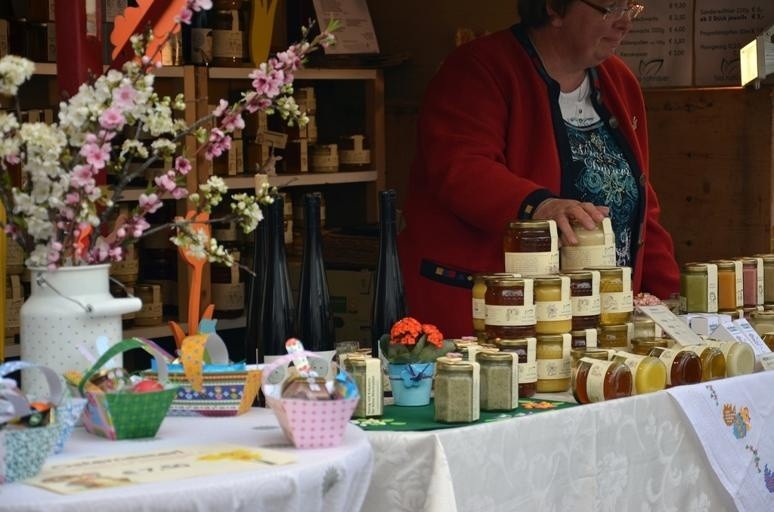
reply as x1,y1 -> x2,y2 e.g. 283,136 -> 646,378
0,316 -> 774,512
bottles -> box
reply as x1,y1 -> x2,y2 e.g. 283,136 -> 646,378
246,190 -> 408,408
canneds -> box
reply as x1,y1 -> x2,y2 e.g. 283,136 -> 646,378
755,254 -> 774,303
532,277 -> 573,335
207,86 -> 370,176
648,346 -> 703,387
500,338 -> 538,397
573,349 -> 608,366
737,256 -> 766,307
484,276 -> 536,341
611,350 -> 665,392
570,360 -> 629,403
564,217 -> 614,269
569,271 -> 603,331
470,274 -> 487,329
476,353 -> 519,414
750,311 -> 774,338
598,324 -> 620,346
149,2 -> 247,67
741,306 -> 766,320
502,222 -> 560,274
433,356 -> 480,423
676,343 -> 723,382
712,258 -> 746,311
598,267 -> 629,326
540,335 -> 571,391
718,311 -> 743,320
702,340 -> 753,379
631,338 -> 669,354
680,262 -> 718,314
6,227 -> 165,348
344,357 -> 385,419
573,329 -> 598,350
209,191 -> 328,313
633,316 -> 664,339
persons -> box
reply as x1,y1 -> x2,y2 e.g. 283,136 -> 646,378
395,0 -> 682,339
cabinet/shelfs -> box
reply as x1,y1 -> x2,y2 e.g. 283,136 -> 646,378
0,63 -> 385,356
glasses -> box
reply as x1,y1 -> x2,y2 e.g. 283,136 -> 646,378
584,0 -> 645,23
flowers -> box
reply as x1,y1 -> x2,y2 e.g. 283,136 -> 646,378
380,316 -> 457,364
0,0 -> 339,273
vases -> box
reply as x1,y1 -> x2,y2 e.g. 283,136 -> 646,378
19,265 -> 142,407
384,363 -> 435,406
